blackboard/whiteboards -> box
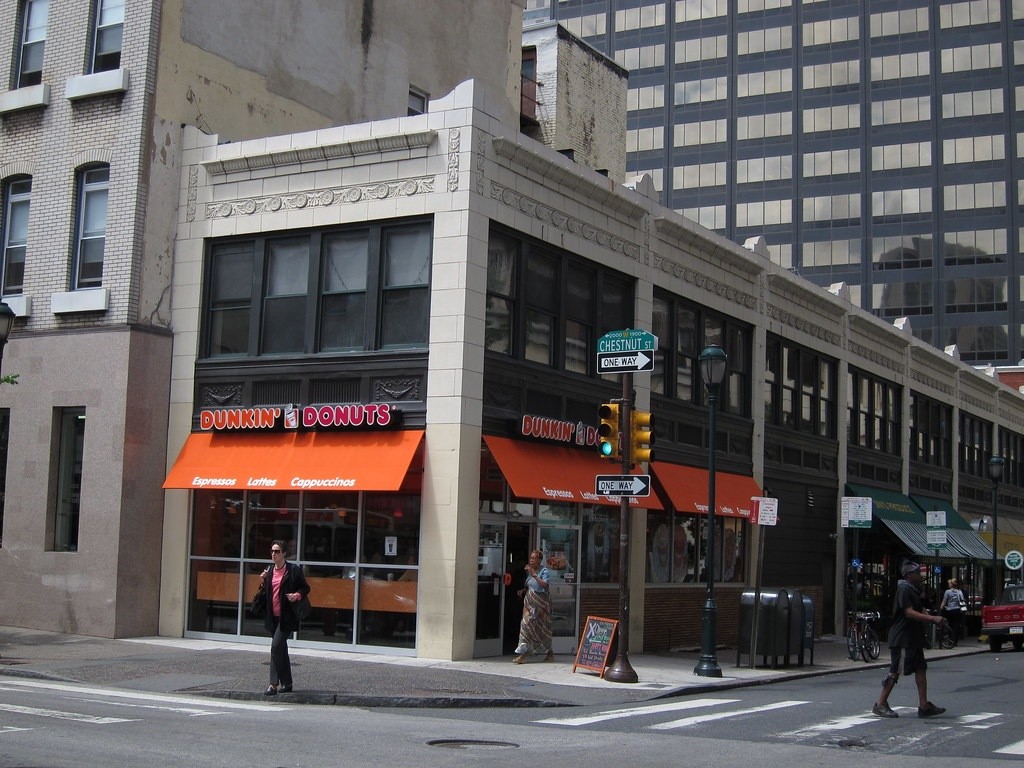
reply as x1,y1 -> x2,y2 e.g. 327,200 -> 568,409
575,615 -> 618,672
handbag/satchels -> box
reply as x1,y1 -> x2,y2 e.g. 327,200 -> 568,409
291,563 -> 313,621
250,566 -> 271,618
957,589 -> 967,614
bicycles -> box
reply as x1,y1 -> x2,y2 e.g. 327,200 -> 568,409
846,609 -> 880,662
920,607 -> 959,650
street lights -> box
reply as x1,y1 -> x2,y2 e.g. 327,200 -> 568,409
987,452 -> 1004,608
693,342 -> 728,678
1,299 -> 16,361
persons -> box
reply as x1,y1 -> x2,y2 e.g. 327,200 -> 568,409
259,540 -> 311,695
848,566 -> 870,602
940,578 -> 969,645
512,550 -> 554,663
318,540 -> 418,640
872,561 -> 946,718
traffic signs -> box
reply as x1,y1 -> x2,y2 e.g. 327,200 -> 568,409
597,349 -> 655,375
594,473 -> 650,497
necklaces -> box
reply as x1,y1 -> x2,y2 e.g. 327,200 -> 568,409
274,562 -> 286,571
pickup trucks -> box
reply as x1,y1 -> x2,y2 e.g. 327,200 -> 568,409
980,584 -> 1024,652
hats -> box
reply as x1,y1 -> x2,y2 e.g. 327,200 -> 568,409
901,558 -> 919,577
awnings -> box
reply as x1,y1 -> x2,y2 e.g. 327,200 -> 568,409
483,434 -> 779,520
845,484 -> 1005,559
163,430 -> 424,491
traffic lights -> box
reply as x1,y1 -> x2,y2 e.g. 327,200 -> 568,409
630,407 -> 655,466
597,402 -> 619,458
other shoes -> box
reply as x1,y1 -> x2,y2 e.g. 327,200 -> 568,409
918,701 -> 945,718
513,657 -> 525,663
278,685 -> 292,693
264,686 -> 277,696
543,656 -> 553,663
872,701 -> 899,718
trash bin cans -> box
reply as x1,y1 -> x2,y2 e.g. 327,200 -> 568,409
738,585 -> 816,657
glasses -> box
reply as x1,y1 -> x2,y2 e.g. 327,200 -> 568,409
271,550 -> 283,553
